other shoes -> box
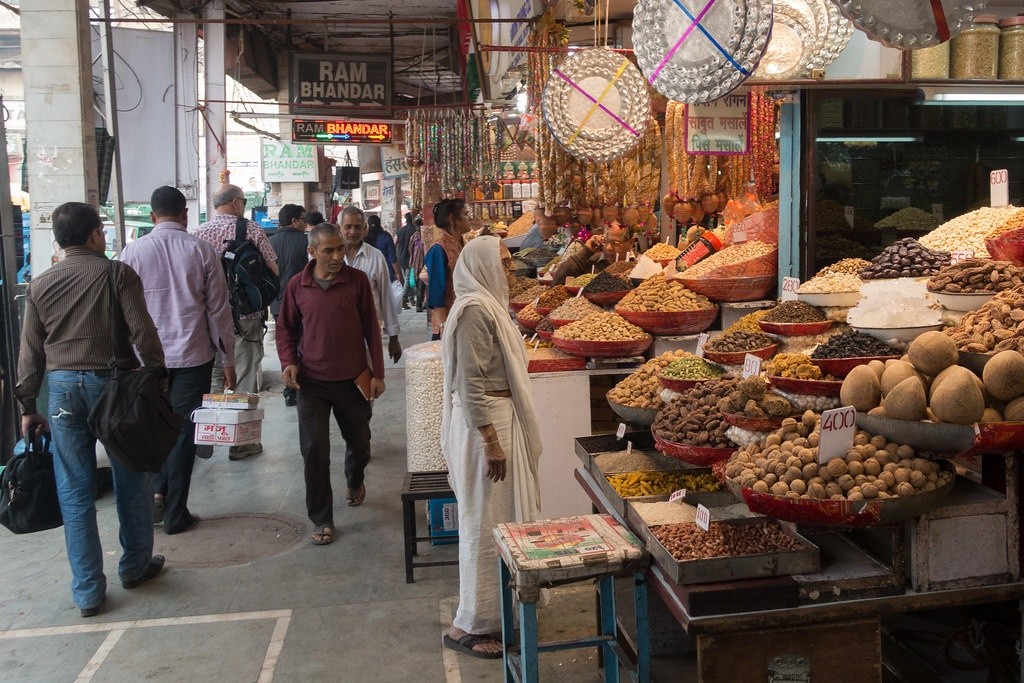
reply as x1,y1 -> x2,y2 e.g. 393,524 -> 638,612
403,295 -> 427,313
228,441 -> 262,460
152,498 -> 164,524
195,444 -> 214,459
285,395 -> 296,406
122,554 -> 165,590
186,512 -> 200,530
80,606 -> 98,617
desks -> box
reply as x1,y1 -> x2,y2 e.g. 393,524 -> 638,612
401,472 -> 469,584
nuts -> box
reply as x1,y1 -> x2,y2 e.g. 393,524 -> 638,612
649,521 -> 805,559
724,408 -> 954,500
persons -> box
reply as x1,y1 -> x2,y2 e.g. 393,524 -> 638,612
439,235 -> 544,659
116,184 -> 279,535
275,222 -> 387,545
549,221 -> 643,281
338,206 -> 402,373
395,209 -> 424,312
424,197 -> 473,341
518,205 -> 544,251
268,204 -> 324,409
13,202 -> 168,618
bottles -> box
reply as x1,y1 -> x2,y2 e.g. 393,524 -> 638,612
899,11 -> 1024,81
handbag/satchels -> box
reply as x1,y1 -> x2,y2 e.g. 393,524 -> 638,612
419,265 -> 429,286
86,370 -> 182,476
409,266 -> 416,288
0,427 -> 65,535
336,149 -> 359,189
391,280 -> 402,314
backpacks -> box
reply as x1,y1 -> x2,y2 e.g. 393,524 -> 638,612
222,217 -> 280,314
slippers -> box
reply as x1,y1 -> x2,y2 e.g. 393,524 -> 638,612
443,632 -> 504,660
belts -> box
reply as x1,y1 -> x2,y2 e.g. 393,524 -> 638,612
80,371 -> 125,379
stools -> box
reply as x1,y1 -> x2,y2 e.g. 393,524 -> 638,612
495,513 -> 651,683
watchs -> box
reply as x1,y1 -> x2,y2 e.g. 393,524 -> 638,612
440,322 -> 446,327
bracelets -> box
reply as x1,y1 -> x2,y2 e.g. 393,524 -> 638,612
481,440 -> 499,444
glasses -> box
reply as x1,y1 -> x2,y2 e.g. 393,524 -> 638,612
502,255 -> 516,269
601,238 -> 628,248
230,196 -> 248,205
291,216 -> 307,224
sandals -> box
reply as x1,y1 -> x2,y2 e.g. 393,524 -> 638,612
344,482 -> 366,506
310,523 -> 335,544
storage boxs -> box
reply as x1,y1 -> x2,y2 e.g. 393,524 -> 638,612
193,395 -> 264,446
